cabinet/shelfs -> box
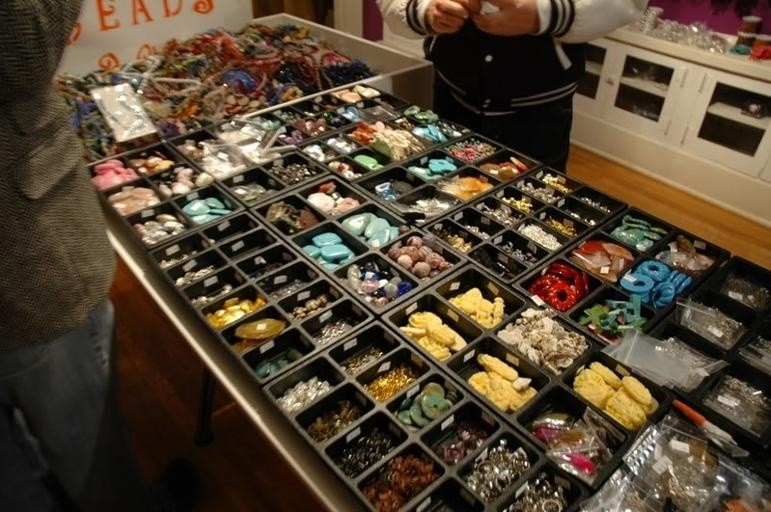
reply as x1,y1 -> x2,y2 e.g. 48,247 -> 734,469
689,65 -> 771,184
555,36 -> 687,153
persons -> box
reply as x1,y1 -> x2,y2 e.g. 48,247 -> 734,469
1,2 -> 121,511
375,0 -> 632,180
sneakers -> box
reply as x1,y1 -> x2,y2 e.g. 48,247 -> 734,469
152,459 -> 200,510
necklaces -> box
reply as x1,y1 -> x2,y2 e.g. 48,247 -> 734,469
59,22 -> 375,162
